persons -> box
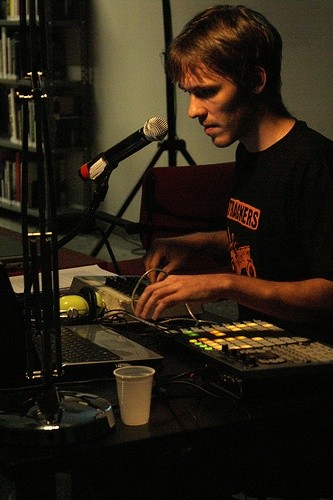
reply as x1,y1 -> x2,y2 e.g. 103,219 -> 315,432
134,4 -> 333,350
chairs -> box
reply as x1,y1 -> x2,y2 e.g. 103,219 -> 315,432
138,163 -> 237,276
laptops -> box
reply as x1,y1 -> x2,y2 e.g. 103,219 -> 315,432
0,264 -> 164,372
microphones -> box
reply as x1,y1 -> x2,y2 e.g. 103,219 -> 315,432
78,117 -> 168,182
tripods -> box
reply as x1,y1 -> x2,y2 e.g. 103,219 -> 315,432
87,0 -> 196,258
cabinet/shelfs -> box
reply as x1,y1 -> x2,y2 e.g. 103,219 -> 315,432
0,0 -> 92,235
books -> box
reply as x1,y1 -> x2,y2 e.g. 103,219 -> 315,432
0,0 -> 40,22
0,26 -> 28,80
0,86 -> 37,147
0,150 -> 41,209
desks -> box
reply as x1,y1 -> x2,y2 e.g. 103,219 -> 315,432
0,226 -> 333,471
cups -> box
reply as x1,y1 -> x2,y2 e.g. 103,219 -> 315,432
113,366 -> 155,426
